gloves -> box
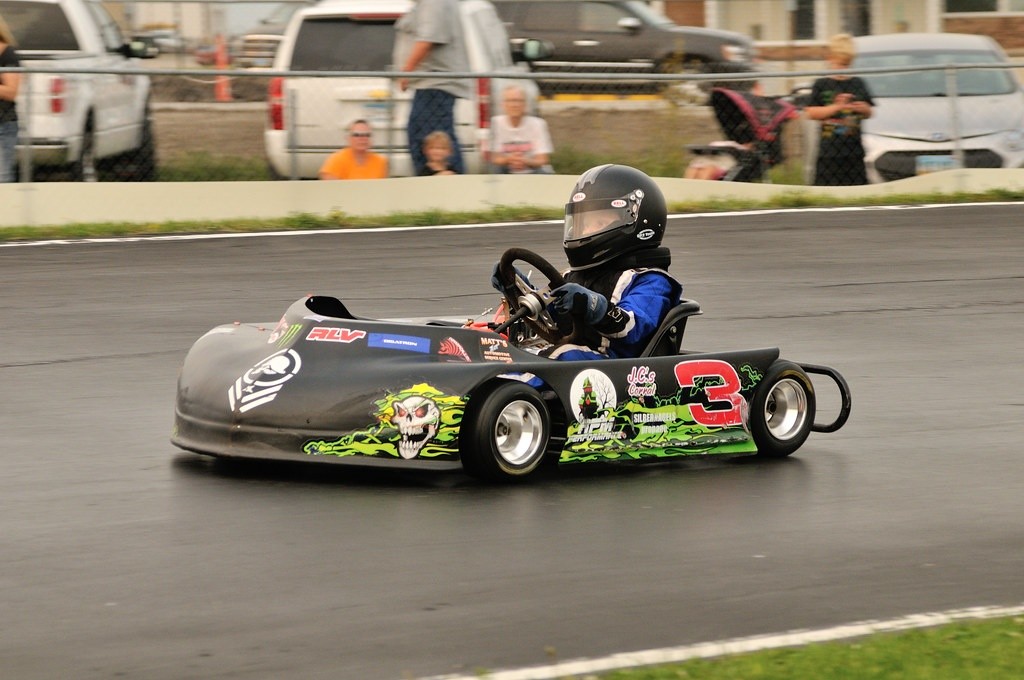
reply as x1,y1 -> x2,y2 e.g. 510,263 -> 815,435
492,262 -> 534,296
548,283 -> 607,325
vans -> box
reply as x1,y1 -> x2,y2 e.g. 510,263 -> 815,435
264,1 -> 540,180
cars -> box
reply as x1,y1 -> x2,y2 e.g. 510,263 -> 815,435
852,33 -> 1024,184
493,1 -> 757,103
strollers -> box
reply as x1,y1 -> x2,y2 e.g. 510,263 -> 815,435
684,87 -> 814,183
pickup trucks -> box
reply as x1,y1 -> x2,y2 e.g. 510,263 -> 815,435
1,1 -> 161,183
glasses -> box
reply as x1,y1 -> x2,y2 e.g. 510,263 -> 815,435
352,132 -> 371,137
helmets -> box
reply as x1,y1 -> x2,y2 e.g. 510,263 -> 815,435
562,164 -> 667,271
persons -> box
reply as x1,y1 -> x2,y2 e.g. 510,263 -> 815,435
464,163 -> 683,362
318,119 -> 390,181
482,79 -> 555,174
0,34 -> 21,183
395,0 -> 470,175
805,33 -> 875,185
